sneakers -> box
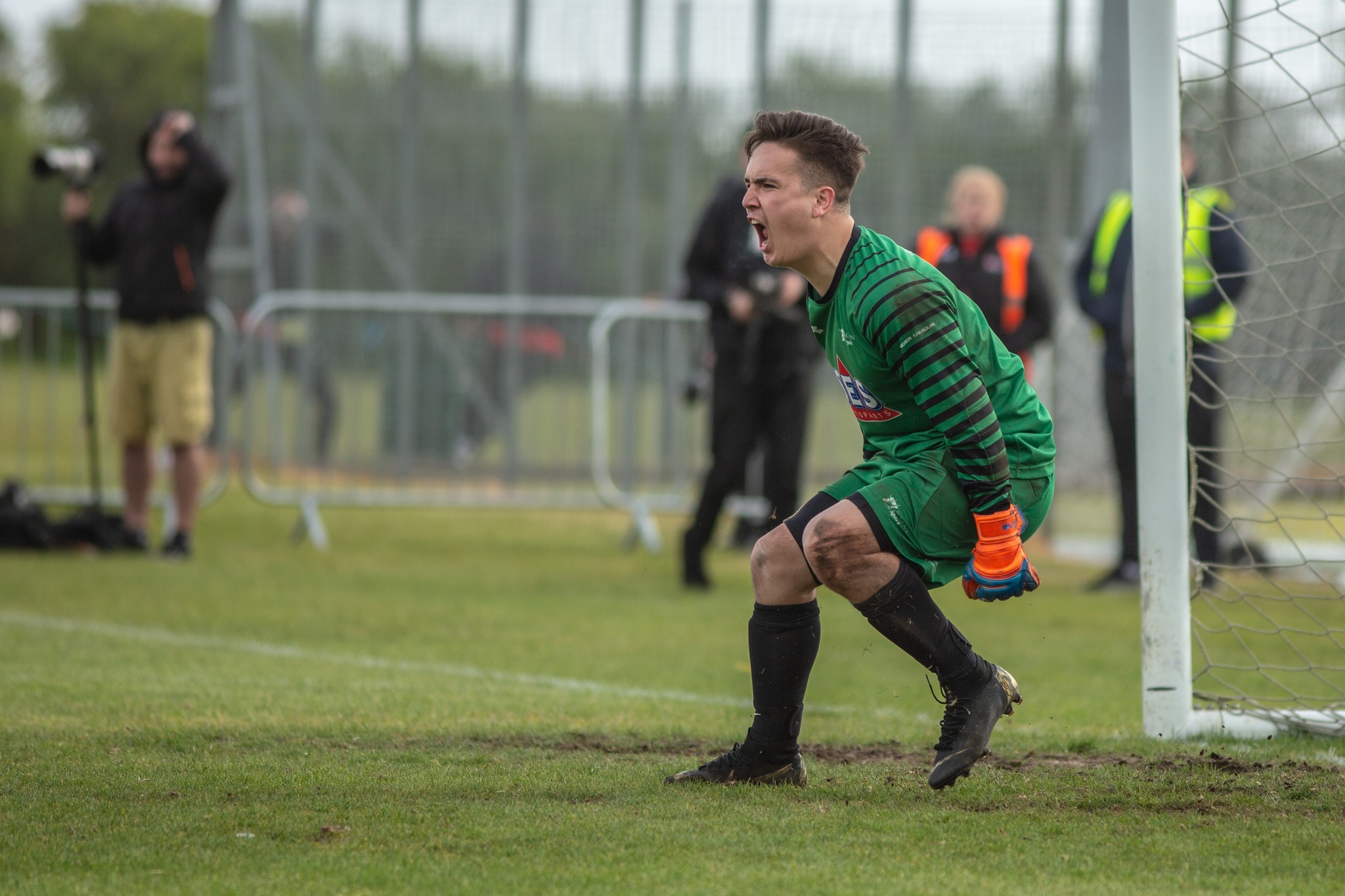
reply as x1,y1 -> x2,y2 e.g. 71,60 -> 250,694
924,653 -> 1022,789
664,729 -> 808,788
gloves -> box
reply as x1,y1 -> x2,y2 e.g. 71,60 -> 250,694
962,501 -> 1041,604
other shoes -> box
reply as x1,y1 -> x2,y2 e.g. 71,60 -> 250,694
164,534 -> 190,554
682,530 -> 710,587
98,529 -> 145,551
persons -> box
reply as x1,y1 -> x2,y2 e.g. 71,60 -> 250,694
58,107 -> 234,555
202,174 -> 340,472
676,110 -> 856,587
903,159 -> 1058,402
448,222 -> 588,478
1066,125 -> 1254,606
657,113 -> 1059,791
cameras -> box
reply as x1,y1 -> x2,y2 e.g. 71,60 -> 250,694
35,145 -> 102,190
